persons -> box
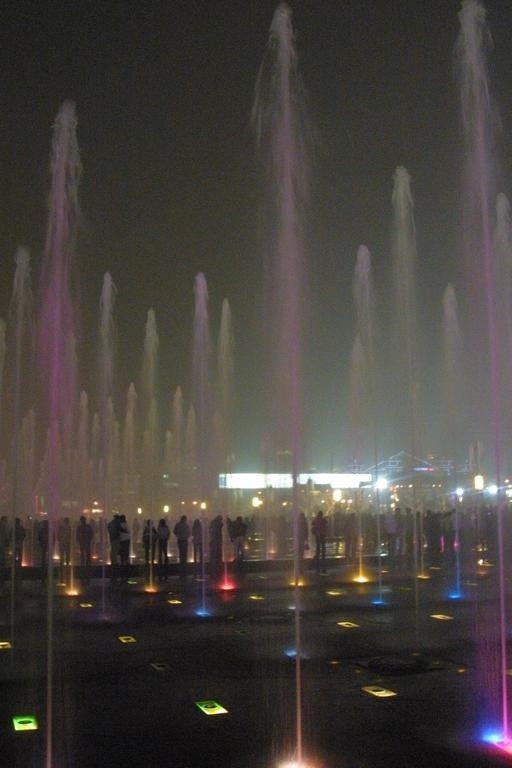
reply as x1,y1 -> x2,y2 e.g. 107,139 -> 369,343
1,507 -> 511,567
